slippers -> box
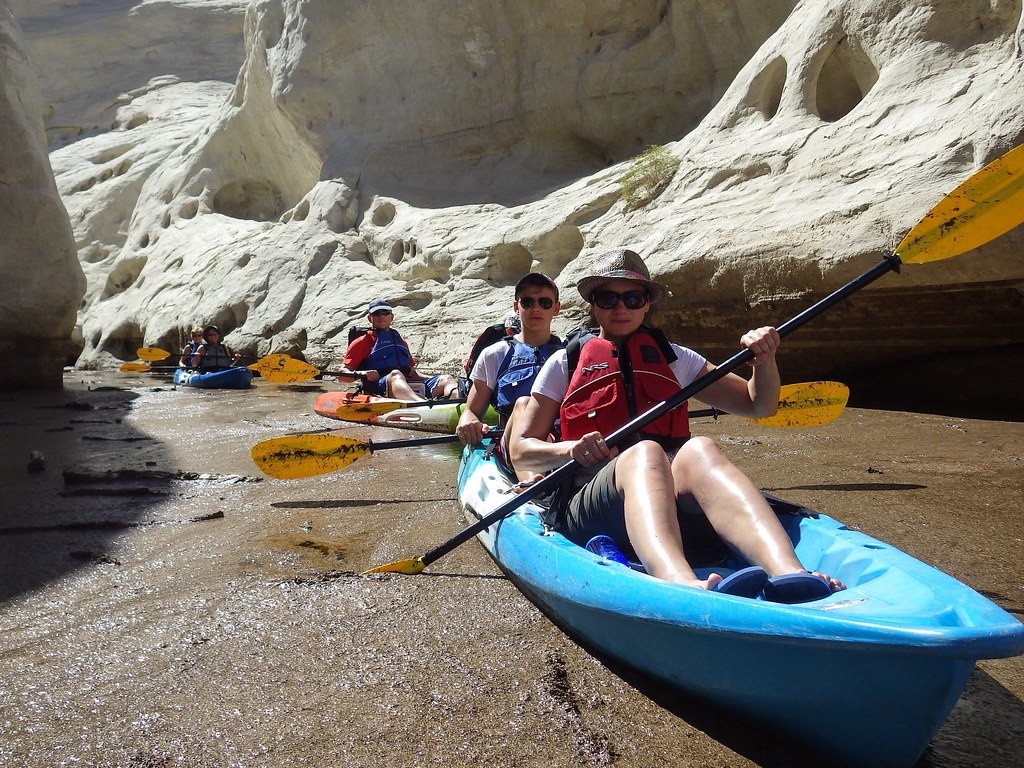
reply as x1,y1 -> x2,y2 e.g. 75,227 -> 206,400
713,567 -> 832,603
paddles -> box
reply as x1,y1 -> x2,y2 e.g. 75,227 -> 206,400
136,348 -> 289,362
254,351 -> 368,385
361,137 -> 1024,577
250,379 -> 852,481
118,359 -> 258,374
334,396 -> 469,421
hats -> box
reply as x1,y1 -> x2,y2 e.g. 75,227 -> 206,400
369,298 -> 392,313
202,325 -> 224,343
577,250 -> 667,306
515,271 -> 559,301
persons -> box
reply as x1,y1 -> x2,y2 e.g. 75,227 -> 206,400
338,300 -> 459,401
465,316 -> 521,395
509,250 -> 847,604
457,272 -> 565,499
179,325 -> 241,375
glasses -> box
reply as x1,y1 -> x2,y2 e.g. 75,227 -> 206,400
193,335 -> 201,337
370,311 -> 390,316
207,333 -> 217,336
519,297 -> 554,310
588,290 -> 649,310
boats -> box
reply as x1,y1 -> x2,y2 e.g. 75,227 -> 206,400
314,391 -> 500,435
454,425 -> 1024,768
172,365 -> 254,389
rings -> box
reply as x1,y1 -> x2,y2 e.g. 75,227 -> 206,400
584,450 -> 590,456
